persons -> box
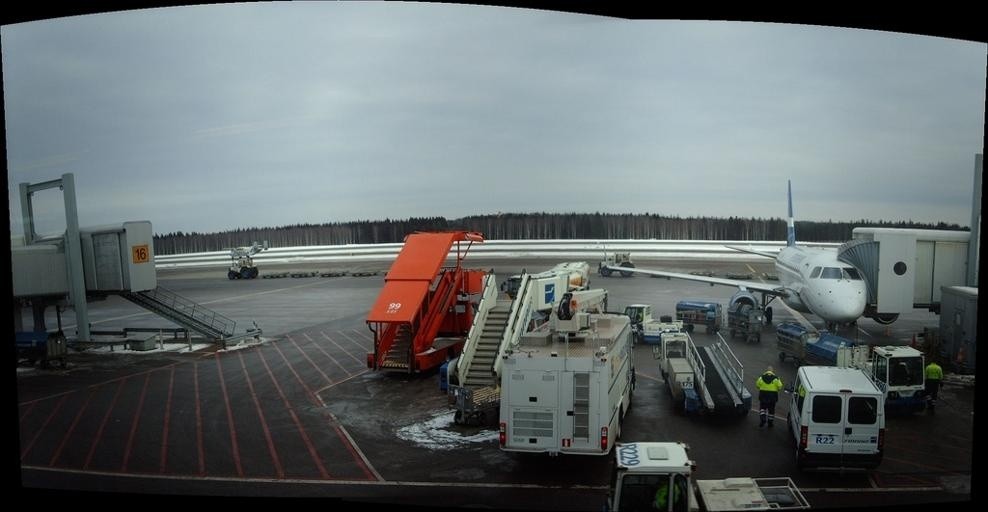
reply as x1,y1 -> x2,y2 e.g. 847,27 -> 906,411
925,357 -> 944,408
756,365 -> 783,429
652,475 -> 681,512
636,308 -> 642,322
797,383 -> 805,414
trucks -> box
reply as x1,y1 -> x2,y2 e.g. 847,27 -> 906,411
621,303 -> 683,343
606,441 -> 811,512
651,331 -> 751,417
775,317 -> 927,416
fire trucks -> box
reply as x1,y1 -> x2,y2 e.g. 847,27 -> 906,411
498,288 -> 636,457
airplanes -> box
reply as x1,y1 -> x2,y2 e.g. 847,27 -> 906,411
606,180 -> 866,332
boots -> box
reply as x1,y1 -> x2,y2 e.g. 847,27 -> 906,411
760,409 -> 774,427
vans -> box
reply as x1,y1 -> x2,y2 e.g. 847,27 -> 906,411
785,366 -> 885,467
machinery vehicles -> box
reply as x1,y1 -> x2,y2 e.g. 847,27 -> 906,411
228,240 -> 269,280
